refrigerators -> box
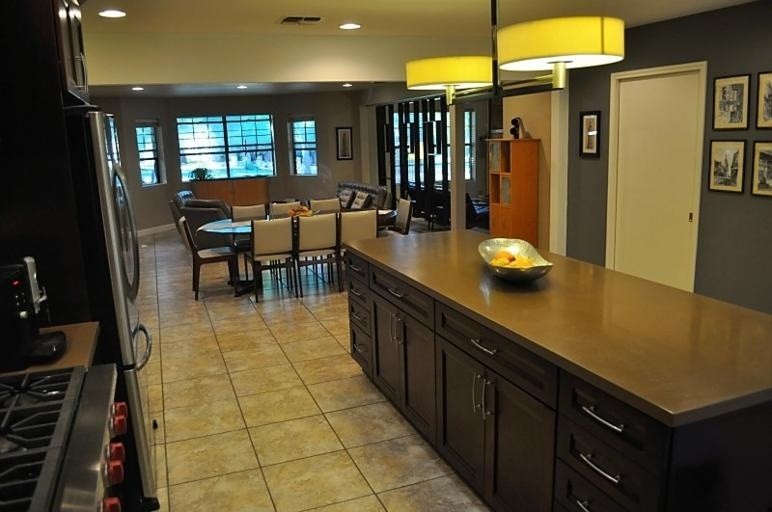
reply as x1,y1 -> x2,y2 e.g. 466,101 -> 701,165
67,99 -> 160,501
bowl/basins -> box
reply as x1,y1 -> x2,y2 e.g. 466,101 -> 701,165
489,130 -> 503,139
478,238 -> 553,287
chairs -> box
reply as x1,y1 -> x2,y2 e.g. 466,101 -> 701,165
157,174 -> 415,305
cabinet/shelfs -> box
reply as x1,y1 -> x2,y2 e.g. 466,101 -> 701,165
554,365 -> 772,512
368,260 -> 438,455
482,135 -> 541,246
342,246 -> 378,382
431,298 -> 556,512
0,0 -> 91,106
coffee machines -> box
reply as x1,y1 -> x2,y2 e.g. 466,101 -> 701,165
1,252 -> 69,373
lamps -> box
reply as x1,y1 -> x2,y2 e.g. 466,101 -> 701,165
397,0 -> 627,105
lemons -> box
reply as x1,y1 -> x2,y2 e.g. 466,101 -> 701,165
496,251 -> 513,261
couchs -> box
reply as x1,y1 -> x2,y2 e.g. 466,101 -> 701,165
405,181 -> 489,229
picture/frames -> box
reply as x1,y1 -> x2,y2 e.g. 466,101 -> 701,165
751,138 -> 772,197
334,125 -> 354,161
753,69 -> 772,131
710,72 -> 753,131
578,108 -> 601,157
706,137 -> 748,196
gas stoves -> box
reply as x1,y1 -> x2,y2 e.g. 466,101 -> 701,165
0,365 -> 131,511
